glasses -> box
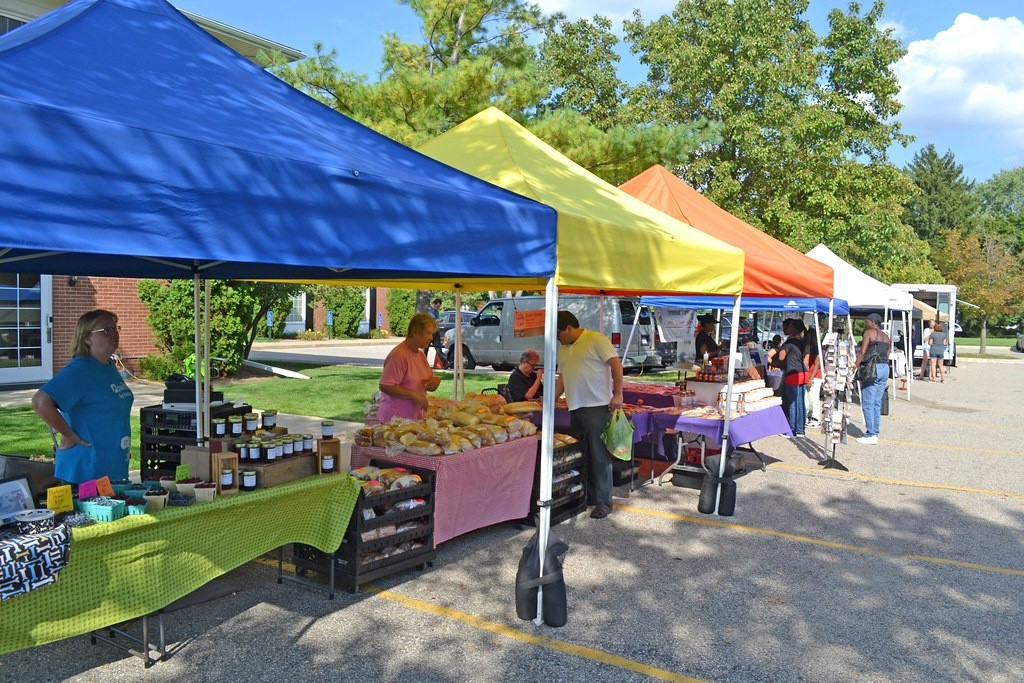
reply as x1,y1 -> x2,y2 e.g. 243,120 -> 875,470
89,326 -> 122,336
524,361 -> 538,368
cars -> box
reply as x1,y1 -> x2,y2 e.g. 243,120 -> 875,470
1016,330 -> 1024,352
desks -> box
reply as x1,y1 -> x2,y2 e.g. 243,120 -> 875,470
530,371 -> 793,492
0,471 -> 362,668
349,434 -> 538,549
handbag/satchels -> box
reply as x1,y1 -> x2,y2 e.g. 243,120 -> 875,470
856,351 -> 879,382
605,408 -> 634,461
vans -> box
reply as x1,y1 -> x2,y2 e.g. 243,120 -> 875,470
442,296 -> 677,375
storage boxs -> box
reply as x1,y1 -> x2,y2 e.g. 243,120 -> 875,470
0,454 -> 60,505
611,460 -> 640,486
317,438 -> 341,475
75,452 -> 239,521
687,447 -> 721,466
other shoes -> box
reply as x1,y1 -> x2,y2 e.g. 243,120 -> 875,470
779,431 -> 804,438
914,376 -> 923,380
856,435 -> 878,444
806,417 -> 821,428
941,380 -> 945,384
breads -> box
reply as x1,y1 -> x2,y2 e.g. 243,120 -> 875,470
350,466 -> 427,563
352,392 -> 578,456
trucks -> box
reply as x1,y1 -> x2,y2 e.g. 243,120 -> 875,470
888,284 -> 980,366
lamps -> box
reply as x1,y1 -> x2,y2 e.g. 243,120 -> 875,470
69,276 -> 78,286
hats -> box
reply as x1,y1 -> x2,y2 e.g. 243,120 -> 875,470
701,315 -> 720,325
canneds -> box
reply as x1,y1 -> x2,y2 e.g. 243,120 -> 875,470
211,409 -> 334,491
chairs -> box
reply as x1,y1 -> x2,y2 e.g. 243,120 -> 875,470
481,384 -> 514,403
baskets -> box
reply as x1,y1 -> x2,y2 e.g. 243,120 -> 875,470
70,475 -> 219,523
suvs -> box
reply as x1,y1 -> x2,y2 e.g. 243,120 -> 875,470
429,311 -> 483,346
697,312 -> 789,355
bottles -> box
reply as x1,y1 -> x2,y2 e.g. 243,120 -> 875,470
637,400 -> 643,407
322,421 -> 334,441
739,334 -> 759,350
235,441 -> 262,464
262,410 -> 277,429
734,371 -> 749,382
714,371 -> 728,382
709,356 -> 728,372
228,416 -> 242,437
211,419 -> 225,438
675,381 -> 687,391
282,434 -> 313,458
244,412 -> 258,435
221,469 -> 234,489
238,470 -> 256,491
703,351 -> 708,366
717,392 -> 727,410
696,369 -> 713,382
737,394 -> 746,413
679,391 -> 695,397
253,430 -> 283,463
322,456 -> 334,473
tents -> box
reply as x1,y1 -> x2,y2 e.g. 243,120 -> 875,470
0,1 -> 557,622
458,163 -> 835,466
805,243 -> 911,402
616,297 -> 866,426
206,106 -> 742,512
848,300 -> 948,380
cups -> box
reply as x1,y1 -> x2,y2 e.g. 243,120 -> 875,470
747,343 -> 753,348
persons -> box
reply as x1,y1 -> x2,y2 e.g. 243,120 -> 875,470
32,310 -> 134,487
768,335 -> 784,369
424,299 -> 450,370
929,324 -> 947,383
508,350 -> 543,402
916,319 -> 935,381
555,311 -> 624,519
779,313 -> 835,437
379,313 -> 441,423
854,313 -> 891,444
696,315 -> 725,365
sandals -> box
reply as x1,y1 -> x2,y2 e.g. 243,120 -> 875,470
590,501 -> 613,518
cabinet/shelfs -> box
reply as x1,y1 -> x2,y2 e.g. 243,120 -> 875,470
291,459 -> 437,593
138,402 -> 251,481
521,426 -> 588,529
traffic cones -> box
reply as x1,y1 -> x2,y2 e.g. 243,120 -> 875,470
433,352 -> 443,368
